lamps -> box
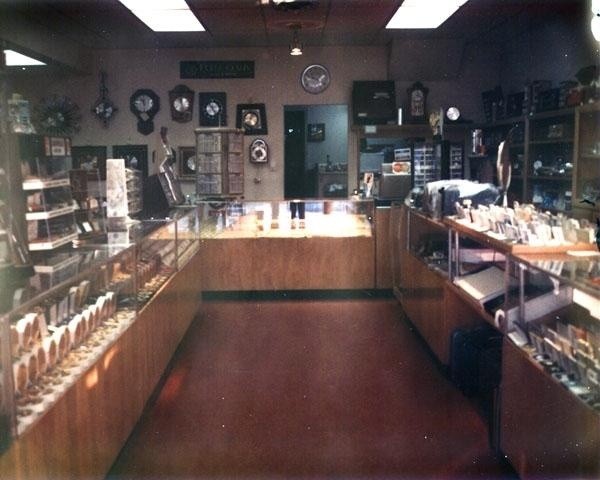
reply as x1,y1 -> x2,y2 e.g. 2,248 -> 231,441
289,25 -> 303,56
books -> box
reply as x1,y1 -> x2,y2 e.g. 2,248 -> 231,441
453,266 -> 525,309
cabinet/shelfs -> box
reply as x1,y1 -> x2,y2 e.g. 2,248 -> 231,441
22,175 -> 84,291
465,103 -> 600,222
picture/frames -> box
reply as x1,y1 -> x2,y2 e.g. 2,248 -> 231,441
307,123 -> 325,143
70,145 -> 107,181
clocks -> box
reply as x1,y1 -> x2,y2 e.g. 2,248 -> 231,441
406,82 -> 461,124
92,70 -> 270,182
301,64 -> 330,95
